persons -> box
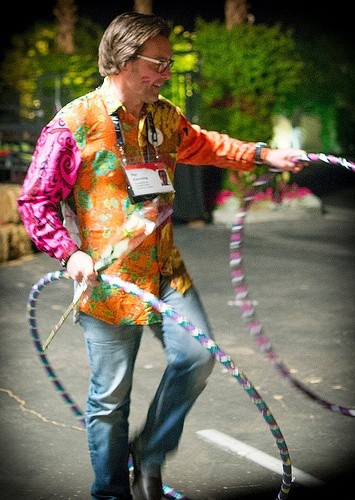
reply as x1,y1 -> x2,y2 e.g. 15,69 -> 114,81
158,170 -> 170,186
16,12 -> 308,500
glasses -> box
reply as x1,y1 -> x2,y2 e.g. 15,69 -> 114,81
133,53 -> 175,75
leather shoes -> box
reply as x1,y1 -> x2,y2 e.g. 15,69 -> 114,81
129,437 -> 167,500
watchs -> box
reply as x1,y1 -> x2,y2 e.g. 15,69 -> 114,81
254,141 -> 269,163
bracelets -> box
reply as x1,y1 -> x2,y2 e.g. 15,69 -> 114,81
61,248 -> 80,267
